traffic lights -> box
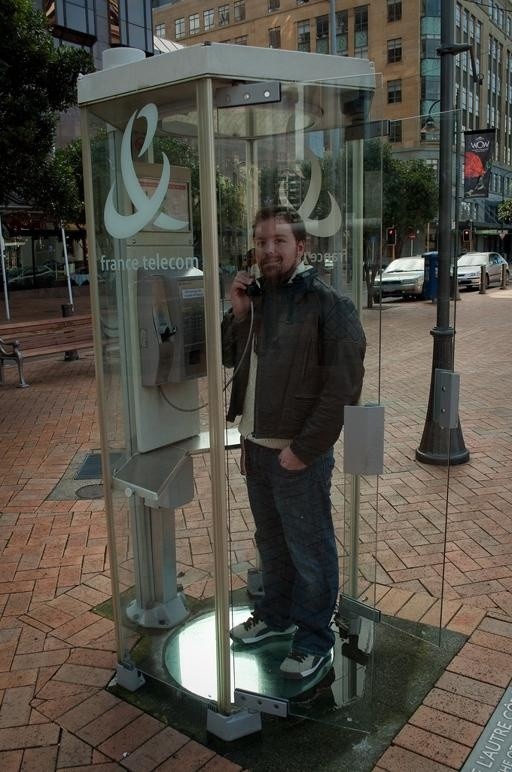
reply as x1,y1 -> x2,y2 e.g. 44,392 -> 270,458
385,227 -> 396,246
463,229 -> 470,242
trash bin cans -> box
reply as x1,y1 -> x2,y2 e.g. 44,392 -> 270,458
421,251 -> 439,300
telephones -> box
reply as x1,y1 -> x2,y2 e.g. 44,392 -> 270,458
246,248 -> 263,296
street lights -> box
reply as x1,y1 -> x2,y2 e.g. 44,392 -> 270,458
419,87 -> 463,300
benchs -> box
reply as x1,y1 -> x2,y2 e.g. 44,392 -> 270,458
0,310 -> 118,388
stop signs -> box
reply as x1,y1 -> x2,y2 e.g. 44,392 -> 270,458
408,230 -> 417,240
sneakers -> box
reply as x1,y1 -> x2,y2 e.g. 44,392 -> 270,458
229,616 -> 297,644
279,646 -> 331,680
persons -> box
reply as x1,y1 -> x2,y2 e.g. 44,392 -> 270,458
217,202 -> 369,685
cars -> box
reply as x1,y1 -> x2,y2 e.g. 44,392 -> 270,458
370,254 -> 455,304
323,257 -> 335,272
455,251 -> 510,290
5,265 -> 55,291
